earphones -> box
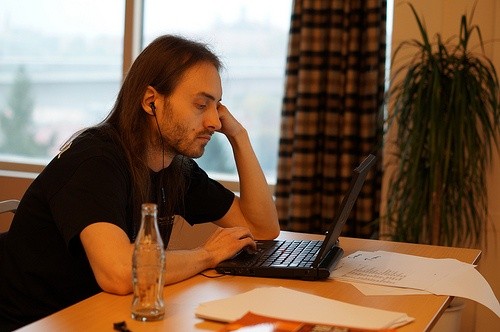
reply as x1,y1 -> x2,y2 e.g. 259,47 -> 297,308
150,102 -> 156,116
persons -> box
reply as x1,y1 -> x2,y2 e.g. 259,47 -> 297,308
0,32 -> 280,330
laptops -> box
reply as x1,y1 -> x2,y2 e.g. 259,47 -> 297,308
215,154 -> 376,280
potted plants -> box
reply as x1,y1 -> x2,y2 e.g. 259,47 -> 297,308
373,0 -> 499,332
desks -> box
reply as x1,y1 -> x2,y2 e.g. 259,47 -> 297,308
13,231 -> 482,332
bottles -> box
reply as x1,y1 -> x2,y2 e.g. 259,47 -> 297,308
130,203 -> 165,321
